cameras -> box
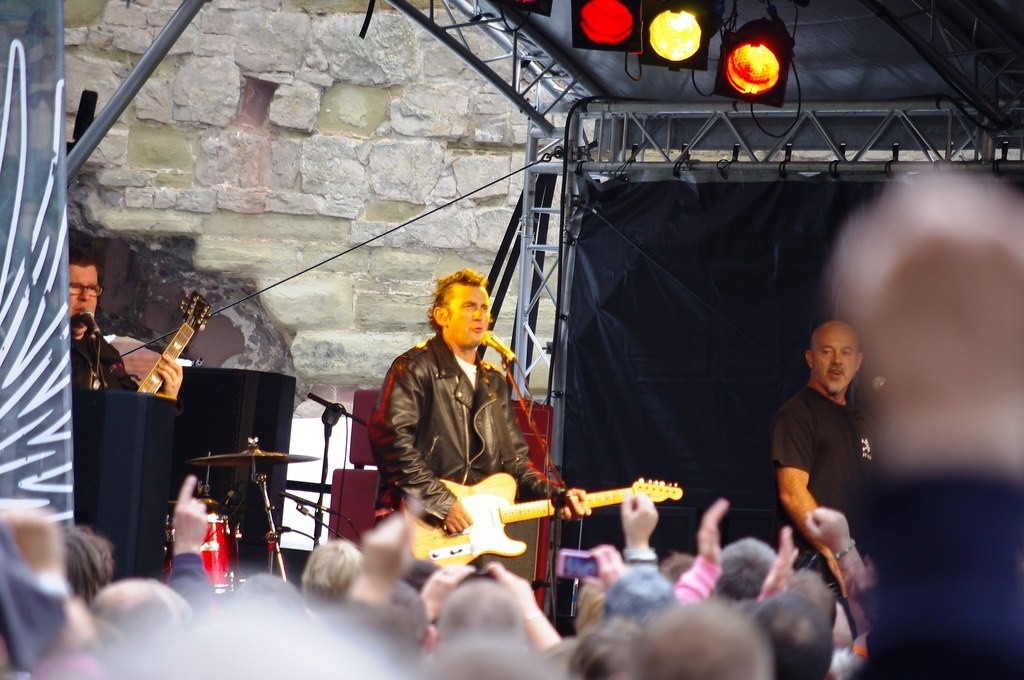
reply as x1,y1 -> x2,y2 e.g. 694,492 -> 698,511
556,548 -> 597,581
462,569 -> 491,583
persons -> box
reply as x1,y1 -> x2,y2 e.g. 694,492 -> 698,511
367,267 -> 592,569
772,321 -> 876,640
68,230 -> 184,399
0,489 -> 875,679
838,167 -> 1024,680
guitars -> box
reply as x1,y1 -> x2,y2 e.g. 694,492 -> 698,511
136,290 -> 211,394
403,472 -> 684,569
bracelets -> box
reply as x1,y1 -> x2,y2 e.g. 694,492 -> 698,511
836,543 -> 853,559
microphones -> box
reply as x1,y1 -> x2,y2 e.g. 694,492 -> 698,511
79,308 -> 100,334
480,331 -> 516,362
279,489 -> 336,516
871,377 -> 889,392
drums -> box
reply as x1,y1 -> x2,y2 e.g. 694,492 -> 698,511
163,511 -> 240,594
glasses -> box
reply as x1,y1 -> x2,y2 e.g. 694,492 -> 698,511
69,282 -> 103,296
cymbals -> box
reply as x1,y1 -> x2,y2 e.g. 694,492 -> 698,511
167,496 -> 221,507
188,448 -> 322,466
